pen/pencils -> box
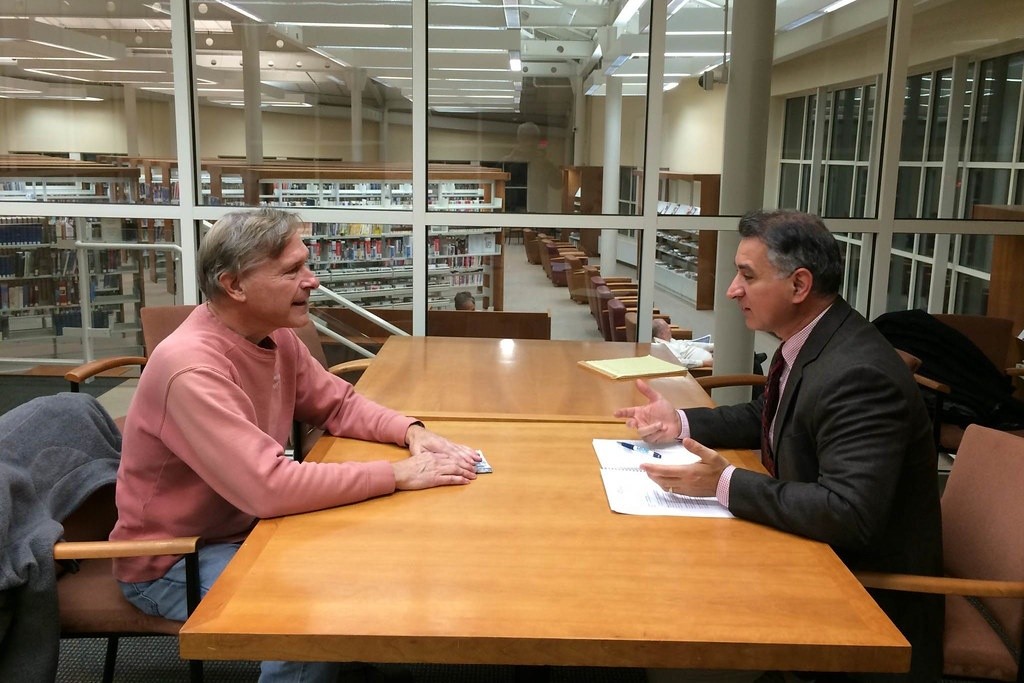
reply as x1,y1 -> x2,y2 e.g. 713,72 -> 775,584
618,441 -> 662,459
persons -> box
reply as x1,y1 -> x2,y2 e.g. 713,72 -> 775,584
497,121 -> 568,215
611,208 -> 950,683
453,289 -> 477,312
647,316 -> 770,401
106,198 -> 485,683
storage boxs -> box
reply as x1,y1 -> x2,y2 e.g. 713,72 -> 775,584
5,315 -> 56,337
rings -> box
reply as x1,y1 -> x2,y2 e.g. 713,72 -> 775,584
667,487 -> 673,494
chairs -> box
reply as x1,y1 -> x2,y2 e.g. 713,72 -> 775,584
291,319 -> 371,461
850,422 -> 1024,682
60,304 -> 199,438
0,390 -> 202,682
692,347 -> 922,388
915,314 -> 1023,455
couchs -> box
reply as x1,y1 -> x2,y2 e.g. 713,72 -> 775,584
523,227 -> 714,376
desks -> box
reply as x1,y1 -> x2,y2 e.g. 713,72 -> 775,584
176,419 -> 912,682
350,334 -> 718,424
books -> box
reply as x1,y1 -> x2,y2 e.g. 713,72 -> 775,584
0,169 -> 498,337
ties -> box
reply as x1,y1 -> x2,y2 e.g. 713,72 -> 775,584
760,341 -> 786,479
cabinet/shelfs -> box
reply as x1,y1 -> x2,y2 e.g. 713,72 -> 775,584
130,158 -> 511,310
566,165 -> 603,256
655,172 -> 720,313
0,167 -> 146,375
